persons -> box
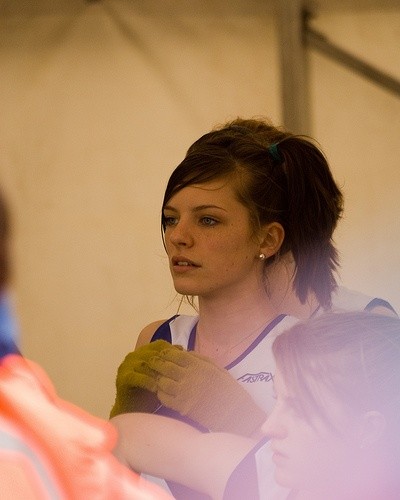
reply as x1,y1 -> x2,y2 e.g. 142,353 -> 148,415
108,117 -> 400,500
0,163 -> 178,500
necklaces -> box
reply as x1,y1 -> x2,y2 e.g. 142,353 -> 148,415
196,315 -> 279,360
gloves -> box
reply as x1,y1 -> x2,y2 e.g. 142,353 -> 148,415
145,345 -> 264,437
109,339 -> 187,420
